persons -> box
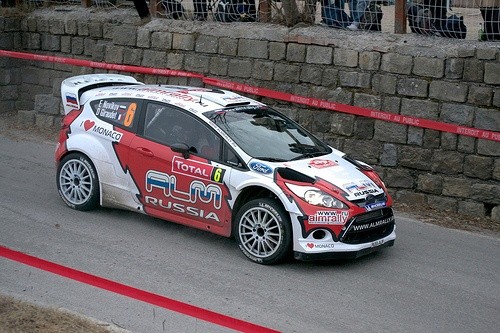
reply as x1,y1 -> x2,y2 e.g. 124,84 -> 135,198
159,0 -> 498,42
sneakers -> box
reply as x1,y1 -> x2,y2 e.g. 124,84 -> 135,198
347,24 -> 358,31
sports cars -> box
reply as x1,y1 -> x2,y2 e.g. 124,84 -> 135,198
55,73 -> 397,266
